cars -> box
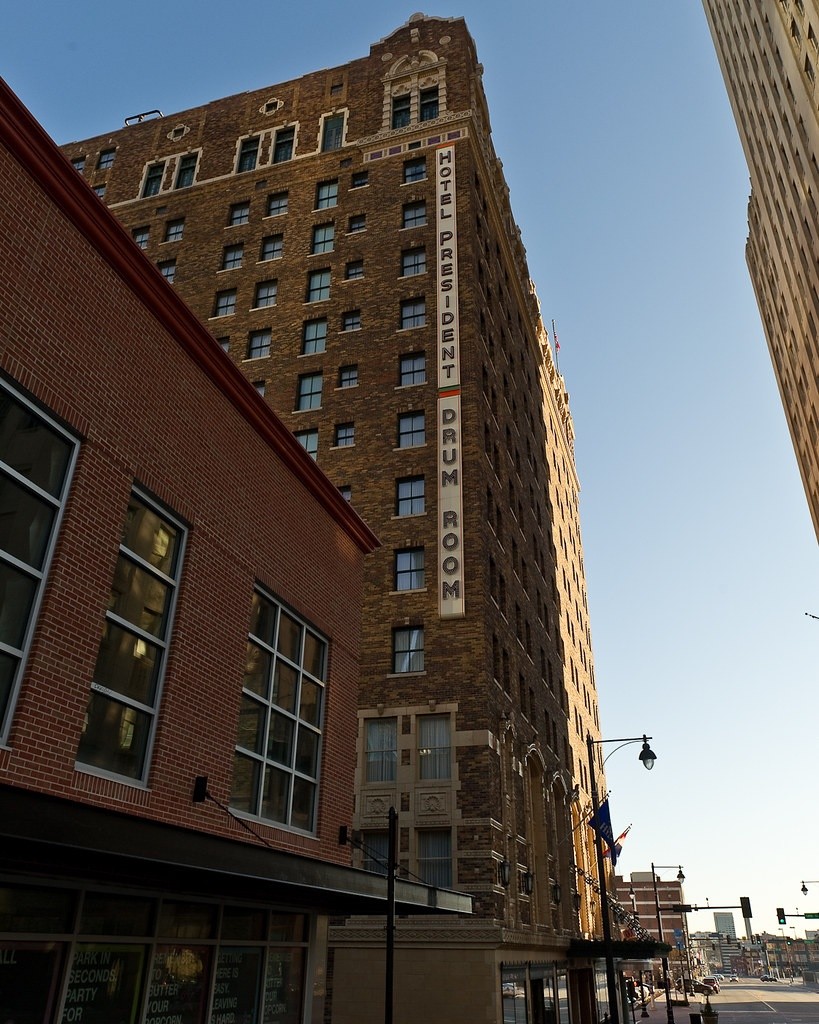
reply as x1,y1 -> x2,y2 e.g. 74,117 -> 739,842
729,975 -> 739,982
712,974 -> 724,980
760,975 -> 777,983
625,981 -> 655,1003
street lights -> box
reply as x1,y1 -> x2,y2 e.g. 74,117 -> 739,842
628,882 -> 650,1018
651,863 -> 686,1024
586,733 -> 657,1024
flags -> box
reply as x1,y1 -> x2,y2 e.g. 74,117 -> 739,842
587,798 -> 617,867
600,832 -> 626,867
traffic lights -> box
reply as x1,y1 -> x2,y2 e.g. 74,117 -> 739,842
776,908 -> 786,925
757,936 -> 761,944
702,976 -> 720,994
787,937 -> 791,946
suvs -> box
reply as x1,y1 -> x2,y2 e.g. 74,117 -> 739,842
675,978 -> 713,996
